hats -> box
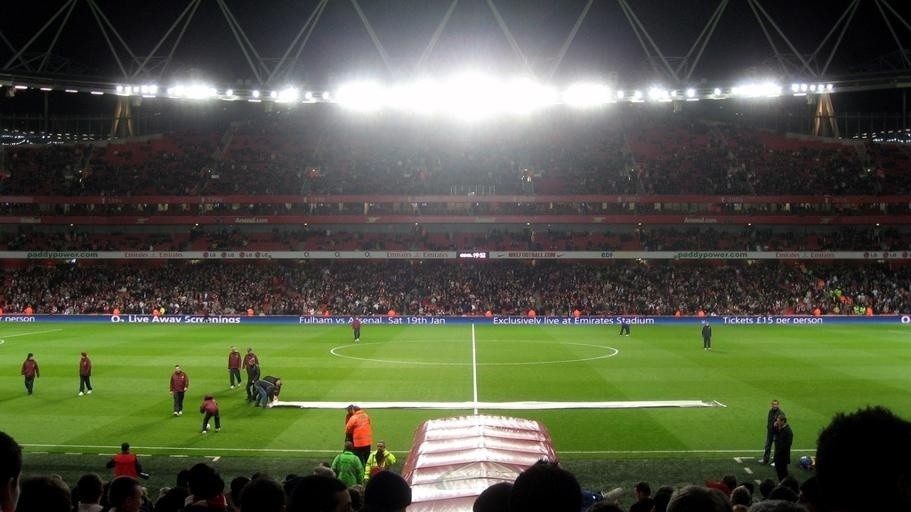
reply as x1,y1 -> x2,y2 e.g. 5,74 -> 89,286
344,404 -> 360,413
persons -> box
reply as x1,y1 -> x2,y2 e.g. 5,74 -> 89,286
702,321 -> 713,351
472,458 -> 813,511
169,364 -> 190,416
225,439 -> 412,511
2,104 -> 911,321
263,375 -> 282,400
619,312 -> 631,337
254,378 -> 281,409
199,393 -> 221,435
242,347 -> 261,375
814,403 -> 911,511
757,401 -> 793,481
344,404 -> 372,463
21,352 -> 40,397
0,431 -> 238,512
352,315 -> 361,342
246,358 -> 261,403
78,351 -> 93,396
228,344 -> 243,389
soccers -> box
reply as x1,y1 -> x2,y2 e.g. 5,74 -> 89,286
206,424 -> 210,430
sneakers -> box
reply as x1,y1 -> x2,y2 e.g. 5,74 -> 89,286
230,383 -> 242,389
173,410 -> 183,417
78,390 -> 93,397
245,396 -> 268,408
201,427 -> 220,435
